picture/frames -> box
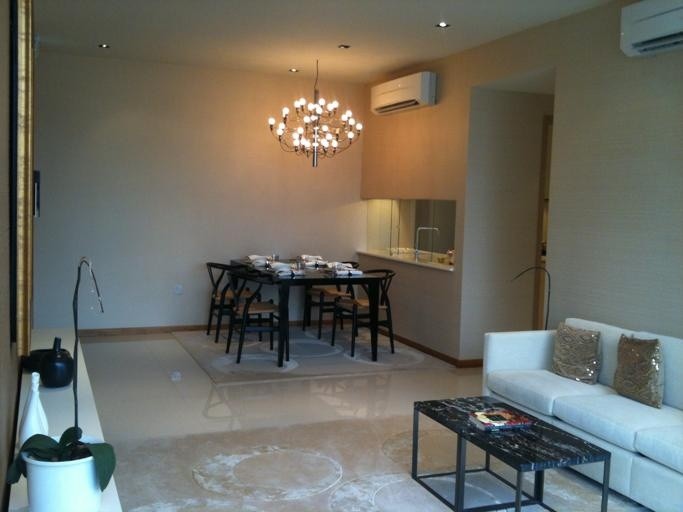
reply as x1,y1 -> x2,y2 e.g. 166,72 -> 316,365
10,2 -> 33,357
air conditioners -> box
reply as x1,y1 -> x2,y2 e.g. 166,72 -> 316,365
620,1 -> 683,57
370,70 -> 437,118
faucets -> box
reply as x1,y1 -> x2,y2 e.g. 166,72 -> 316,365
413,224 -> 442,262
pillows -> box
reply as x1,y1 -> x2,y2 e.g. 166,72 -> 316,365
614,332 -> 664,409
551,323 -> 600,386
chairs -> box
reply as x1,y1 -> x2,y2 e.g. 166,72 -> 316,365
225,271 -> 292,364
206,261 -> 263,343
302,262 -> 359,339
331,269 -> 395,356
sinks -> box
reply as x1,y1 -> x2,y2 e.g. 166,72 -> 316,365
396,247 -> 446,266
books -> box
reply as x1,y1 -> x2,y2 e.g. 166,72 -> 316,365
468,406 -> 532,431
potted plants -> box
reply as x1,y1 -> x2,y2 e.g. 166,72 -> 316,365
6,427 -> 117,511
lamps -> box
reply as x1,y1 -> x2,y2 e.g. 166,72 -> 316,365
268,58 -> 364,169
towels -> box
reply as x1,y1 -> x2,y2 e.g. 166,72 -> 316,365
300,251 -> 330,270
328,259 -> 352,270
270,254 -> 296,271
336,265 -> 363,278
275,267 -> 307,280
246,252 -> 272,272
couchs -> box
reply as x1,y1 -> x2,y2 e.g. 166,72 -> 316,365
482,316 -> 682,512
